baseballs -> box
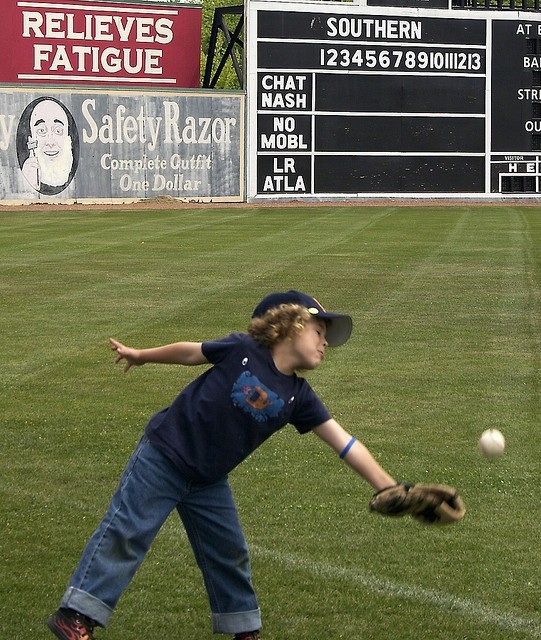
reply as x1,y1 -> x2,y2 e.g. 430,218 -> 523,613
479,428 -> 506,457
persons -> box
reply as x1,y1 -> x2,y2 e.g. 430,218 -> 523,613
20,100 -> 74,191
47,291 -> 467,636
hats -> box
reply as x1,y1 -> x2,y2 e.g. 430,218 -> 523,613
253,291 -> 353,348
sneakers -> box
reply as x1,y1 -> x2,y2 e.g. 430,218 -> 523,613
234,630 -> 261,640
47,609 -> 98,640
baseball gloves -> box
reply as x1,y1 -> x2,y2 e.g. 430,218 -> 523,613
367,483 -> 464,522
